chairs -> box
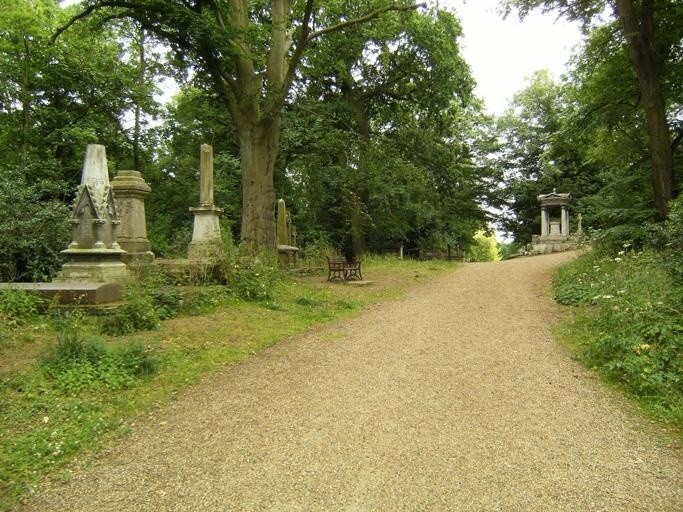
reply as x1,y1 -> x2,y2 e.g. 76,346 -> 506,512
327,254 -> 365,284
